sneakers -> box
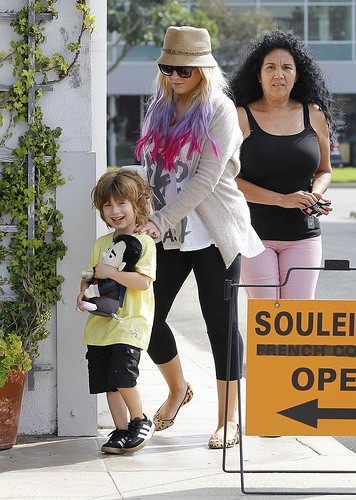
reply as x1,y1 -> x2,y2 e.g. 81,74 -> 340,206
123,413 -> 155,453
101,428 -> 128,454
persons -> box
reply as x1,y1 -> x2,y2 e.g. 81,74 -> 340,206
77,170 -> 157,454
133,26 -> 266,449
229,29 -> 350,299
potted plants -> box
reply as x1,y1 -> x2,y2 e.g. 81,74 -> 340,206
0,0 -> 94,449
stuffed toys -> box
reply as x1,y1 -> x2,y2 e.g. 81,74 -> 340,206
77,233 -> 143,322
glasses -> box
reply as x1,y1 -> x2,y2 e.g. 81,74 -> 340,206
158,64 -> 196,78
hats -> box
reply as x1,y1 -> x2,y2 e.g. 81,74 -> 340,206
155,25 -> 217,67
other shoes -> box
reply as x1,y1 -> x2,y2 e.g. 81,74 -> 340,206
153,380 -> 193,432
208,423 -> 240,449
